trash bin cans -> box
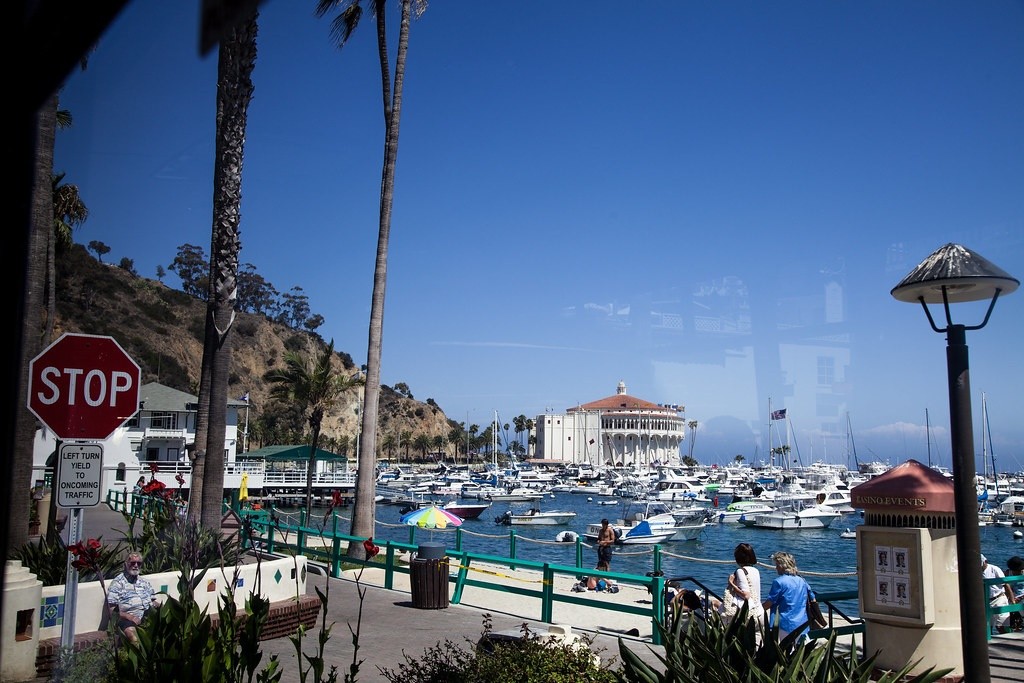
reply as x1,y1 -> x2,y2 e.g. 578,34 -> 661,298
408,544 -> 449,611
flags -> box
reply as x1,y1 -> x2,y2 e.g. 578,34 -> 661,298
771,409 -> 786,420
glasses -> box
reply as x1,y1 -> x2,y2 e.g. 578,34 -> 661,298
128,561 -> 143,566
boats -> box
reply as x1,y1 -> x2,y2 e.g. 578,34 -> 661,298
345,391 -> 1024,529
400,494 -> 487,520
583,495 -> 708,541
495,496 -> 576,525
617,521 -> 678,546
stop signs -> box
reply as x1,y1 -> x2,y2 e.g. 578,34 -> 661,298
26,332 -> 142,441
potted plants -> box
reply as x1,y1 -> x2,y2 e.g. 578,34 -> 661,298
30,505 -> 41,535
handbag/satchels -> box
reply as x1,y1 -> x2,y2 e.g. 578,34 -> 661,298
723,567 -> 757,616
799,574 -> 828,631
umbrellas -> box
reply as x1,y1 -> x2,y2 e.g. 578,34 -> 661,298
239,472 -> 249,511
398,504 -> 465,541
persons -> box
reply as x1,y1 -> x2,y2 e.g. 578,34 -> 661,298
981,553 -> 1016,634
896,553 -> 905,567
761,551 -> 816,650
718,543 -> 764,632
880,583 -> 888,595
106,552 -> 165,650
1002,556 -> 1024,633
134,475 -> 145,517
897,583 -> 906,598
879,553 -> 888,566
595,517 -> 614,572
670,589 -> 721,636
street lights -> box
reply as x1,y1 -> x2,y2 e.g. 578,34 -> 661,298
890,243 -> 1023,682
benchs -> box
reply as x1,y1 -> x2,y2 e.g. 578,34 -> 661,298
35,555 -> 323,676
